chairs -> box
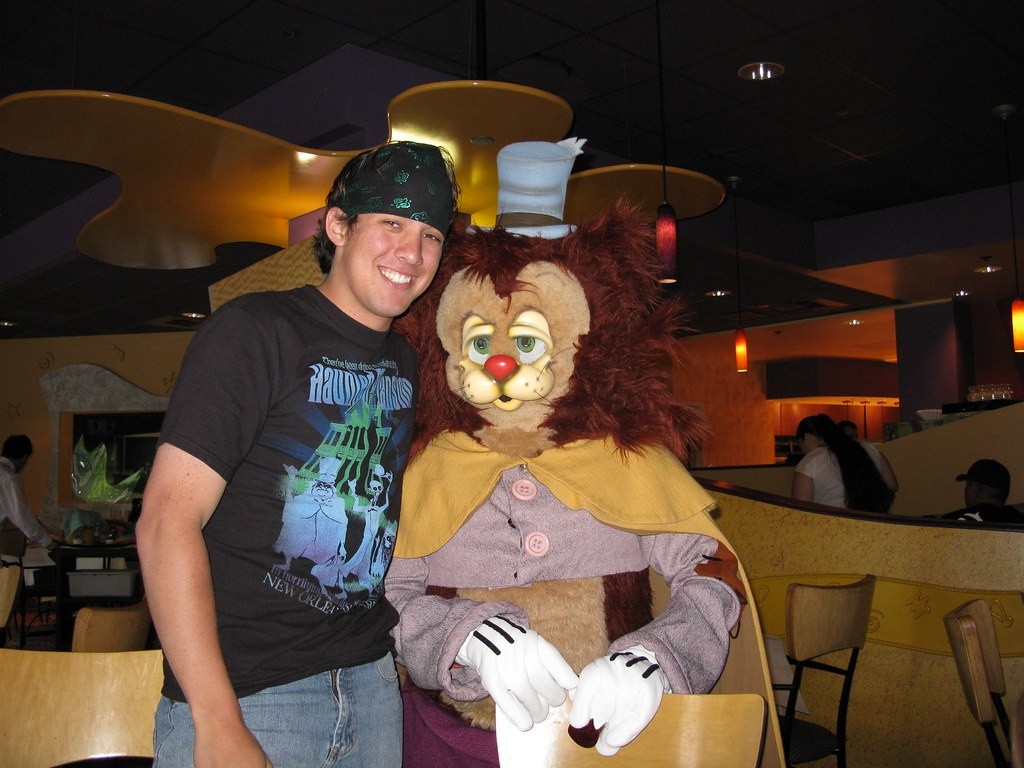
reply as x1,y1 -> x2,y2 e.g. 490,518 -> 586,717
71,599 -> 154,651
770,573 -> 877,768
940,589 -> 1024,768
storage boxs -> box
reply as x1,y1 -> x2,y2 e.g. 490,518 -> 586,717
64,502 -> 142,598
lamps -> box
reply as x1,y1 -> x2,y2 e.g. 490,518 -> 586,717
726,175 -> 750,373
654,0 -> 680,260
990,103 -> 1024,352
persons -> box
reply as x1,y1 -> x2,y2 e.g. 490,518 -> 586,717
136,140 -> 464,768
792,413 -> 1024,528
0,434 -> 62,639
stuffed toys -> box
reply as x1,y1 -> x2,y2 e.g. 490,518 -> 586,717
394,138 -> 784,768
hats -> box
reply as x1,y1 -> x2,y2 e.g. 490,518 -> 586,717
955,459 -> 1010,490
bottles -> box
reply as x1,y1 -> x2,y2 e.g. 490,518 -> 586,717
128,498 -> 143,534
73,519 -> 117,546
967,383 -> 1014,402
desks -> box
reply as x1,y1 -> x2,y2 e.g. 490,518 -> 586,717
58,546 -> 138,569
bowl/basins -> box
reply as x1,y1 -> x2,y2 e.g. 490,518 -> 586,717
916,409 -> 943,420
